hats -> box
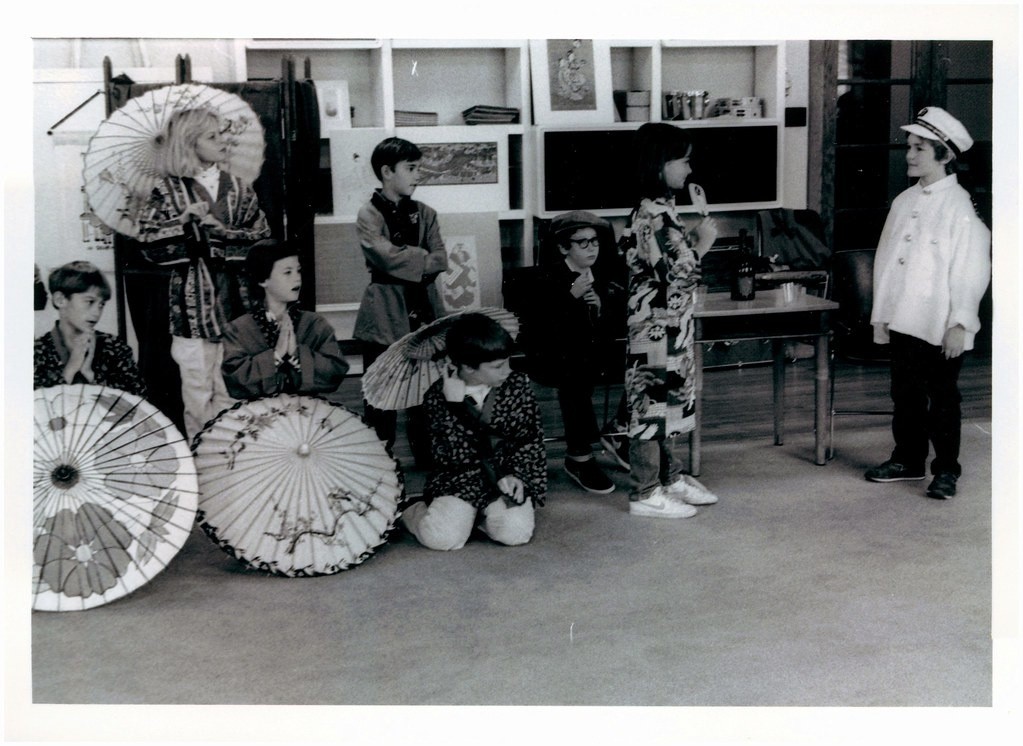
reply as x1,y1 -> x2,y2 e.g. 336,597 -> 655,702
551,208 -> 610,239
899,107 -> 975,159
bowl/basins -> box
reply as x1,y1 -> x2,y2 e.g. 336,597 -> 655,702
662,94 -> 706,120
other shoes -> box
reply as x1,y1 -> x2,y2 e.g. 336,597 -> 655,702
389,491 -> 425,543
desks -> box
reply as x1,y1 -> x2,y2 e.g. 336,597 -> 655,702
690,288 -> 840,477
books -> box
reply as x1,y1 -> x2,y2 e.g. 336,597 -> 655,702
463,106 -> 517,125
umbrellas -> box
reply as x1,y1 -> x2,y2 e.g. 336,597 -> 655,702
32,384 -> 199,613
82,82 -> 266,238
361,306 -> 521,411
189,390 -> 406,576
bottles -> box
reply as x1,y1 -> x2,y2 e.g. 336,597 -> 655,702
729,228 -> 756,301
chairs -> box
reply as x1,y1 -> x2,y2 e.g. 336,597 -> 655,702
503,267 -> 625,442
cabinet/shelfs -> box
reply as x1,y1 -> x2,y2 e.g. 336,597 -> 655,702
232,39 -> 784,375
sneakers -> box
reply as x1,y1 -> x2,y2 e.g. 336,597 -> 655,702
628,487 -> 698,520
663,475 -> 718,505
863,457 -> 927,482
563,454 -> 618,495
925,470 -> 958,499
600,421 -> 632,471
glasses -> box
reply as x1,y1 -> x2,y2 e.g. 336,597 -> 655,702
567,234 -> 601,248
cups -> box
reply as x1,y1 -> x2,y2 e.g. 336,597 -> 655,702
780,282 -> 795,304
693,284 -> 707,307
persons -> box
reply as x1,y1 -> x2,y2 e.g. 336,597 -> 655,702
864,106 -> 991,498
352,137 -> 448,471
137,109 -> 271,448
509,210 -> 630,494
617,122 -> 717,519
402,313 -> 548,551
221,244 -> 350,401
34,260 -> 148,400
